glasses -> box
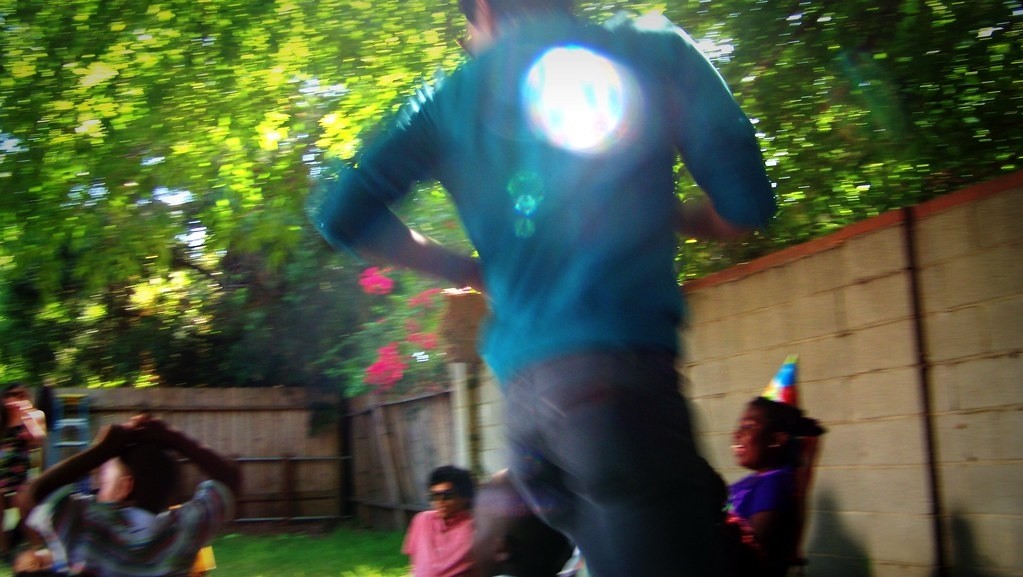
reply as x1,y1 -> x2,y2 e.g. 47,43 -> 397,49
453,8 -> 479,61
426,486 -> 460,502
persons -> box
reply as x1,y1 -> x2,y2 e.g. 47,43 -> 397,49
311,0 -> 779,577
400,354 -> 806,577
0,388 -> 244,577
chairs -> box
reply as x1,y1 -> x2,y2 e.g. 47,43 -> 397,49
731,419 -> 827,577
